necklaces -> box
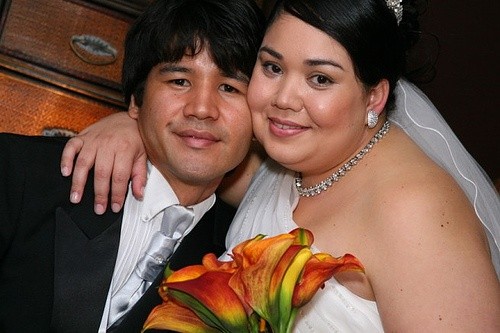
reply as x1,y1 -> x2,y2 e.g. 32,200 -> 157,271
293,121 -> 391,197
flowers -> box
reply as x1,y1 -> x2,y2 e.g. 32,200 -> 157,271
142,227 -> 365,332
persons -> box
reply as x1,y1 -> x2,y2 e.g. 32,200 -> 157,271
1,0 -> 266,333
60,0 -> 500,333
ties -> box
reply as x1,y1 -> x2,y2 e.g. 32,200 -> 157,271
106,204 -> 194,330
